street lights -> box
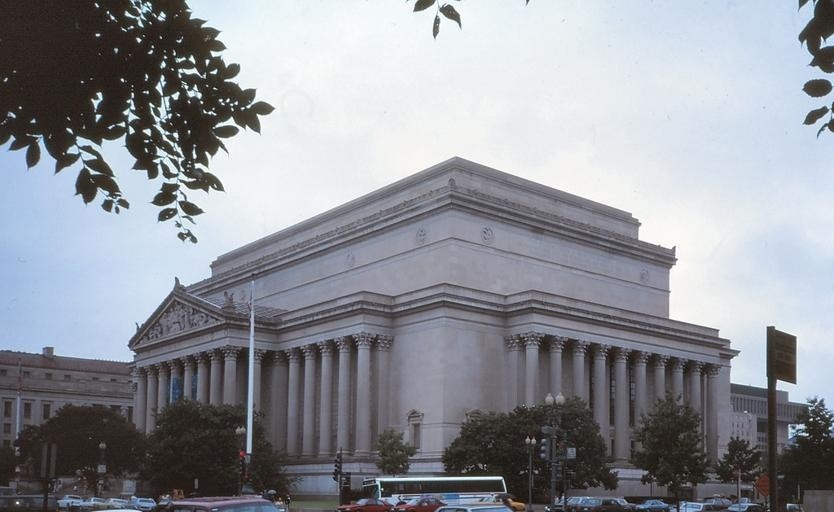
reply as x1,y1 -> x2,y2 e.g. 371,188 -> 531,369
98,441 -> 107,493
235,424 -> 246,495
525,392 -> 567,512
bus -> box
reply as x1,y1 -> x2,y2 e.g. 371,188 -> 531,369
362,475 -> 508,506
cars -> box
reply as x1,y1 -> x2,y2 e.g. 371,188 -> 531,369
545,495 -> 804,512
335,495 -> 515,512
56,494 -> 280,512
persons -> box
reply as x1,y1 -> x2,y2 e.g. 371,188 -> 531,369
255,489 -> 290,510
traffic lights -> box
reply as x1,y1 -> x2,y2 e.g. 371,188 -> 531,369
344,473 -> 351,489
333,458 -> 341,482
